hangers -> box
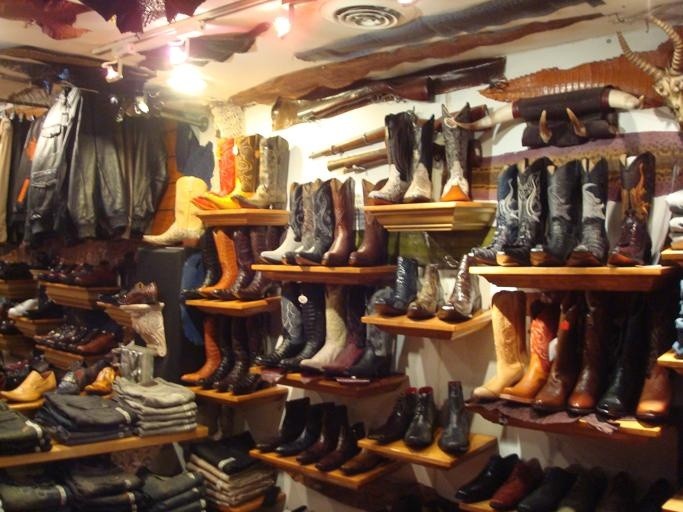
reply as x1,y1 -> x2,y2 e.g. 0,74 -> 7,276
1,93 -> 69,122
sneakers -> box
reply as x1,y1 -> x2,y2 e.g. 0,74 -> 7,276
8,299 -> 39,316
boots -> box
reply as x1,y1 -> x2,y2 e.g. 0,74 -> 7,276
212,225 -> 255,299
259,182 -> 303,264
635,320 -> 672,420
367,112 -> 416,204
567,157 -> 609,266
437,254 -> 480,320
529,159 -> 580,266
228,313 -> 269,394
282,181 -> 313,265
277,285 -> 325,369
298,286 -> 347,373
532,292 -> 583,412
437,381 -> 469,455
343,286 -> 394,378
472,290 -> 526,400
295,405 -> 347,462
294,178 -> 334,266
196,226 -> 239,298
181,226 -> 221,299
374,255 -> 417,314
315,422 -> 365,471
607,151 -> 654,266
180,316 -> 221,385
229,136 -> 289,209
470,163 -> 517,265
256,398 -> 309,451
141,176 -> 207,247
439,102 -> 470,201
595,315 -> 642,418
495,156 -> 553,266
191,138 -> 234,210
407,265 -> 444,317
404,387 -> 435,447
275,402 -> 335,453
321,177 -> 355,266
253,291 -> 305,366
499,300 -> 559,404
212,317 -> 248,391
233,226 -> 278,300
321,286 -> 365,375
198,316 -> 234,387
347,178 -> 388,265
567,292 -> 609,414
197,134 -> 263,209
402,113 -> 434,202
367,388 -> 417,441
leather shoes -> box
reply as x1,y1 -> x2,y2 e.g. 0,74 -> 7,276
47,264 -> 76,282
57,368 -> 80,396
74,266 -> 116,285
85,367 -> 115,395
117,282 -> 158,304
340,448 -> 379,472
517,465 -> 584,512
57,262 -> 92,284
634,478 -> 670,512
99,289 -> 127,304
593,471 -> 634,512
22,301 -> 63,318
67,329 -> 101,351
0,370 -> 57,402
76,332 -> 115,355
553,467 -> 607,512
38,271 -> 49,280
489,458 -> 543,510
455,454 -> 517,500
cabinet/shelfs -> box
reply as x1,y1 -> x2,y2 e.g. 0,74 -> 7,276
1,268 -> 165,382
183,200 -> 683,511
